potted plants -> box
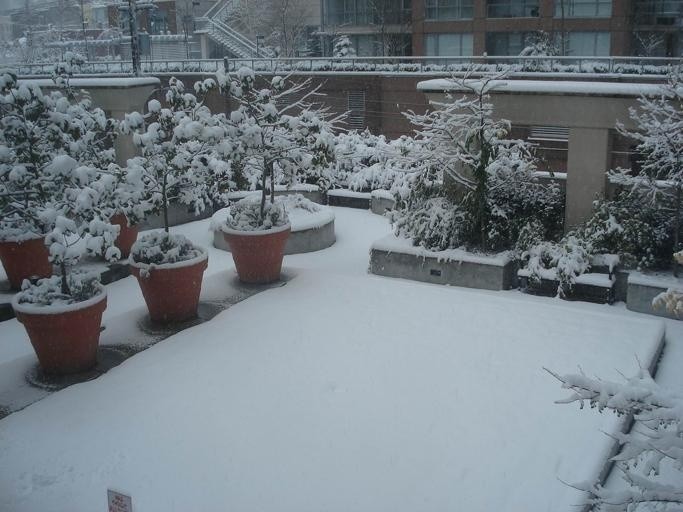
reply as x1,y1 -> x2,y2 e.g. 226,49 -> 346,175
218,67 -> 342,284
0,70 -> 53,290
0,74 -> 123,382
48,52 -> 139,259
110,76 -> 234,324
